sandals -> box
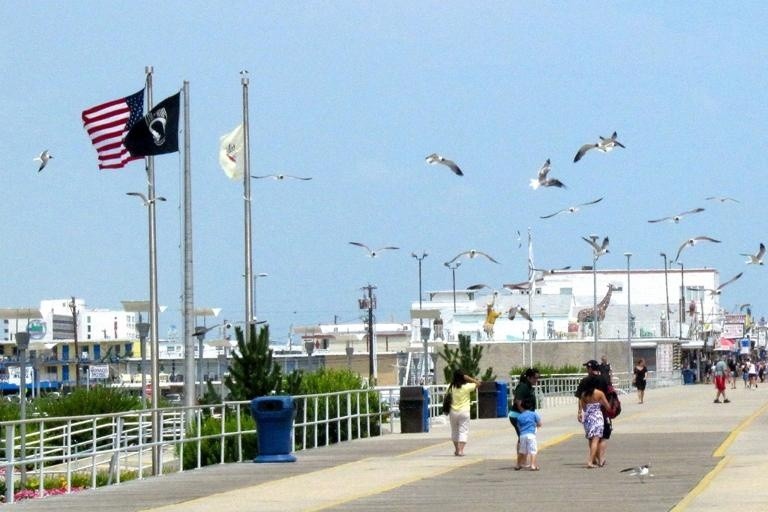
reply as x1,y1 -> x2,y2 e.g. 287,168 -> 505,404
599,459 -> 605,466
588,464 -> 599,468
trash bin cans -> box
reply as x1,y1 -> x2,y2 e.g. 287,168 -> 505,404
397,387 -> 429,434
252,396 -> 299,463
478,381 -> 508,418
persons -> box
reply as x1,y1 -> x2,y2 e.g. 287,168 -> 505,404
517,398 -> 541,470
598,356 -> 611,385
447,369 -> 480,456
763,361 -> 766,375
710,362 -> 716,383
730,365 -> 736,389
634,358 -> 646,405
705,361 -> 710,384
742,363 -> 749,388
578,379 -> 611,469
713,356 -> 730,403
735,362 -> 739,378
575,361 -> 611,439
749,360 -> 758,389
758,362 -> 764,383
508,368 -> 540,468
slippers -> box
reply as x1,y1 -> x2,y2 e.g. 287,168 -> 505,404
515,465 -> 521,470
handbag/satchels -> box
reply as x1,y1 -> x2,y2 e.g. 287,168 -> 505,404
442,383 -> 452,414
632,366 -> 636,386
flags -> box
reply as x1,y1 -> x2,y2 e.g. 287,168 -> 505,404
220,120 -> 249,181
80,90 -> 149,170
126,89 -> 179,159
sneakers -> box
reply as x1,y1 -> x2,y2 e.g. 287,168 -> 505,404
714,400 -> 721,403
523,464 -> 530,468
724,399 -> 730,402
530,466 -> 540,470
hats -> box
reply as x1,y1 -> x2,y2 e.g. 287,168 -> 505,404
583,360 -> 600,367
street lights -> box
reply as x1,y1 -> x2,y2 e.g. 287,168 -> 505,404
1,309 -> 43,492
444,262 -> 462,317
338,333 -> 364,377
252,273 -> 267,320
412,251 -> 427,340
124,301 -> 165,412
193,309 -> 219,401
